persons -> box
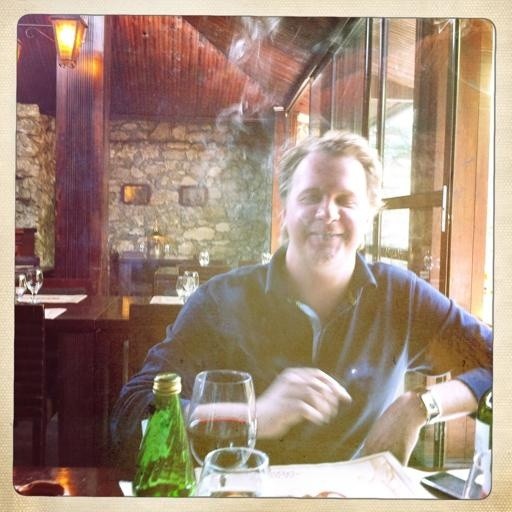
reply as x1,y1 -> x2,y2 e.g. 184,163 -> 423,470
106,128 -> 493,469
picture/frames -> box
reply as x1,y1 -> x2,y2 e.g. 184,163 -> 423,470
179,185 -> 209,207
120,183 -> 151,205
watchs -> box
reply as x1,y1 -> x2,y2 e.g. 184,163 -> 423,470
410,383 -> 441,425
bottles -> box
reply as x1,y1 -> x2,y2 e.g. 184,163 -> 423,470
474,387 -> 493,456
131,371 -> 197,498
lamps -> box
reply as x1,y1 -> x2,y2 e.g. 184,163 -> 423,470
52,15 -> 88,67
17,39 -> 22,62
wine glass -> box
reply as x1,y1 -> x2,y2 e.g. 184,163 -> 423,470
15,273 -> 26,301
463,450 -> 493,499
26,269 -> 44,304
194,447 -> 270,497
175,271 -> 199,303
186,369 -> 257,466
199,252 -> 209,266
